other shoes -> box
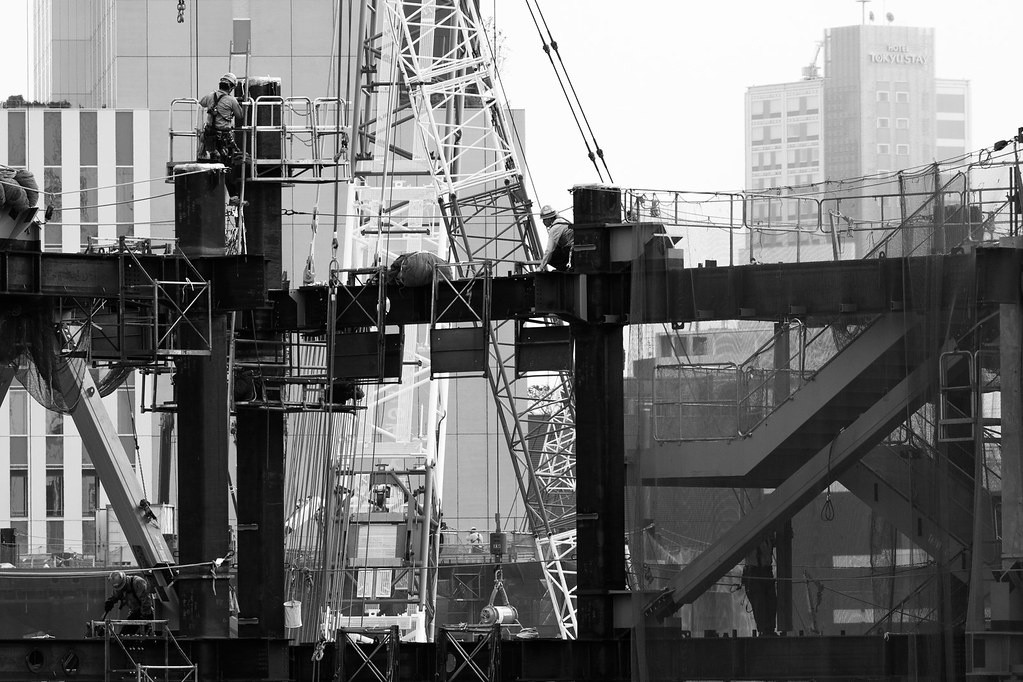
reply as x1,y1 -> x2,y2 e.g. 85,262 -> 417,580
229,198 -> 249,207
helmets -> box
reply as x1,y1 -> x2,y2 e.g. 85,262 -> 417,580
221,73 -> 238,88
540,205 -> 558,219
109,570 -> 127,590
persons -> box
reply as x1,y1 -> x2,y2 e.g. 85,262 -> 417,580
104,570 -> 155,634
536,205 -> 573,271
199,73 -> 249,206
742,529 -> 793,635
466,527 -> 483,553
